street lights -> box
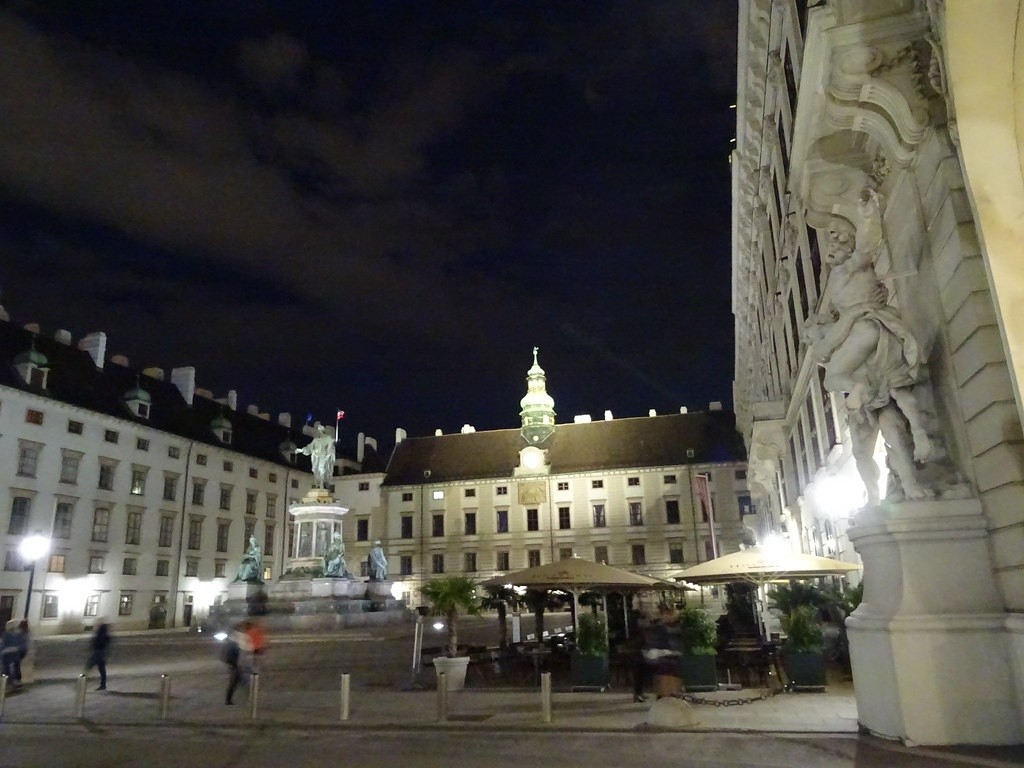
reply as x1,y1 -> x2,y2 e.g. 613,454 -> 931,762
17,530 -> 52,626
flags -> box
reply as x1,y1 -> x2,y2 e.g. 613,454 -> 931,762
337,410 -> 344,420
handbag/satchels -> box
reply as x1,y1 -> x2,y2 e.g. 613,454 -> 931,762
219,640 -> 240,665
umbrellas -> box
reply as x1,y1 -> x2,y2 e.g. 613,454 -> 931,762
663,542 -> 864,641
467,552 -> 698,651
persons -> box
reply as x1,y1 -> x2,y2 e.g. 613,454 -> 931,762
79,622 -> 114,693
236,535 -> 263,581
0,619 -> 29,690
803,187 -> 936,508
224,622 -> 259,706
324,531 -> 347,578
368,538 -> 388,579
294,424 -> 336,489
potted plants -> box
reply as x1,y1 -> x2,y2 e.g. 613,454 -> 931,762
577,611 -> 610,688
416,573 -> 482,691
681,609 -> 721,687
778,603 -> 828,687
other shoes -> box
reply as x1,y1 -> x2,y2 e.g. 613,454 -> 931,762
225,700 -> 234,706
95,686 -> 106,690
